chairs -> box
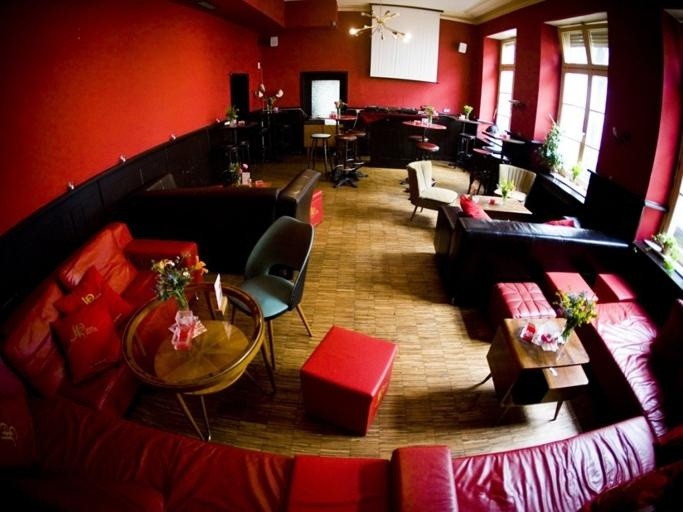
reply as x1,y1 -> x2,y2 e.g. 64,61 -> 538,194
404,157 -> 457,222
494,161 -> 537,205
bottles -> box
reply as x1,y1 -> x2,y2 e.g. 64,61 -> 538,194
241,164 -> 250,185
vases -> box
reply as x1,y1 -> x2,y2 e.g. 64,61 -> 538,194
501,193 -> 506,205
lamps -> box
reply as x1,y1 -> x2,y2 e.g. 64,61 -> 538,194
346,0 -> 408,43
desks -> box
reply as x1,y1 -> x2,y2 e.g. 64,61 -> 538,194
464,194 -> 532,221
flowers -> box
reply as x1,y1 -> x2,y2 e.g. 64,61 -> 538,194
496,173 -> 515,198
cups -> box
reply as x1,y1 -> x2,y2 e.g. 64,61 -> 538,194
414,118 -> 428,126
274,107 -> 278,112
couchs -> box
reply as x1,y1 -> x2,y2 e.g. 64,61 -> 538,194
433,205 -> 639,305
392,300 -> 683,512
1,375 -> 457,512
2,166 -> 322,441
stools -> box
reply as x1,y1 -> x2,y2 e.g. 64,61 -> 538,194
304,114 -> 368,189
299,326 -> 397,438
494,272 -> 637,320
396,107 -> 503,193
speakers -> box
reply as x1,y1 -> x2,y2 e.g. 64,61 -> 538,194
458,42 -> 467,53
271,37 -> 278,46
